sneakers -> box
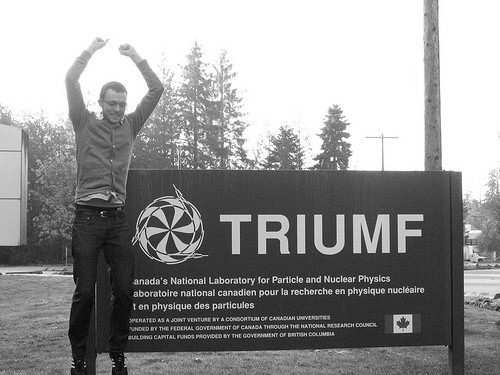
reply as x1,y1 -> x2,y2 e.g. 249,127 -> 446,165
70,356 -> 88,375
108,352 -> 129,375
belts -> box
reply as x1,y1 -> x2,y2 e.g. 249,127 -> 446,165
75,209 -> 127,219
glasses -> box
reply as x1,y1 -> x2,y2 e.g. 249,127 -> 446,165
102,100 -> 126,108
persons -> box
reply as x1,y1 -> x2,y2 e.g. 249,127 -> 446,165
65,36 -> 165,375
